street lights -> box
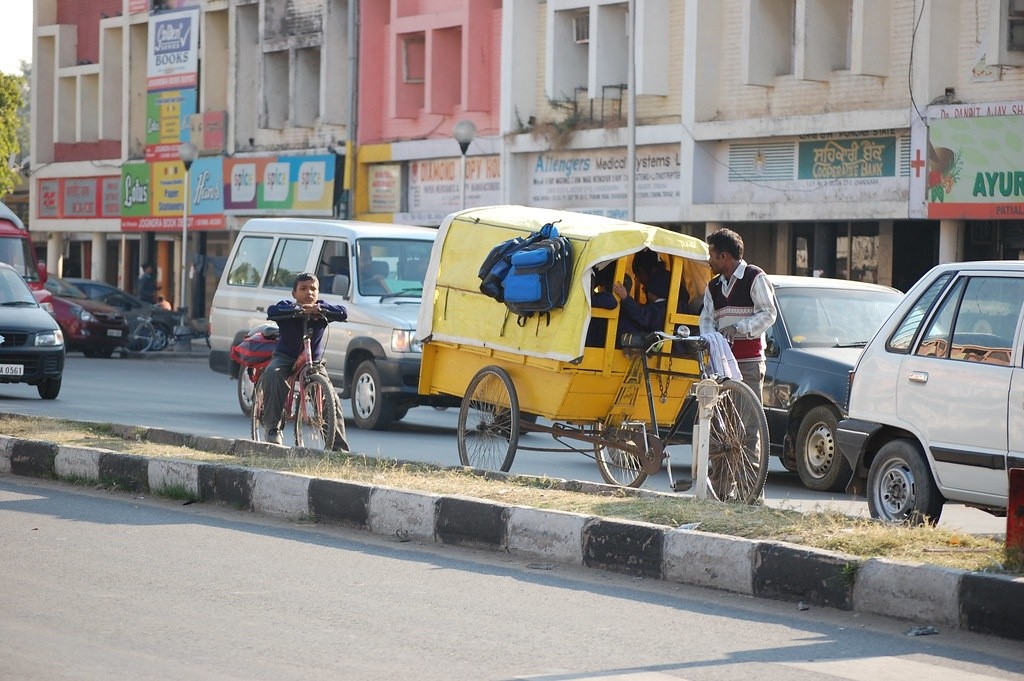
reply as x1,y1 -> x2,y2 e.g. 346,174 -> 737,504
175,142 -> 200,326
452,118 -> 478,212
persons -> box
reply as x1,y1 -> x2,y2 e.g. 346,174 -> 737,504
586,251 -> 692,356
138,265 -> 162,303
326,244 -> 387,295
261,272 -> 350,453
159,296 -> 171,311
699,228 -> 777,506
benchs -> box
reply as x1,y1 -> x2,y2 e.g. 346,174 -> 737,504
932,332 -> 1015,362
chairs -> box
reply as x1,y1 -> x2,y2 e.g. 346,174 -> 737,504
365,261 -> 389,280
328,255 -> 350,294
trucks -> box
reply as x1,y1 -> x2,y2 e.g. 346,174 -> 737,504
0,219 -> 66,339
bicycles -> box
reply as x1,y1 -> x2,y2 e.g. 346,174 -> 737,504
123,302 -> 198,355
236,307 -> 345,452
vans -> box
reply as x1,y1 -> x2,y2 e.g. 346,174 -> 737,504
204,215 -> 464,430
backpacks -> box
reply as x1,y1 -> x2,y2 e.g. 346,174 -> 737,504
478,223 -> 557,304
501,225 -> 573,317
232,334 -> 278,382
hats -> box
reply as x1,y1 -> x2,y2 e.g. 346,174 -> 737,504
633,250 -> 662,275
642,267 -> 670,298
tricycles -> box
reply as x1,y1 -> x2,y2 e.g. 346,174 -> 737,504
415,203 -> 773,505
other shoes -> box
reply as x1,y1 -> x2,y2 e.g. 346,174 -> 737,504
746,500 -> 764,507
716,495 -> 735,504
263,421 -> 284,445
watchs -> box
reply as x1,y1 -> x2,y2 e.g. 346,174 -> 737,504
733,323 -> 738,333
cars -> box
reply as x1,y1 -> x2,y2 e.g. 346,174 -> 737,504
15,265 -> 190,359
0,262 -> 66,399
646,275 -> 949,491
834,258 -> 1024,528
